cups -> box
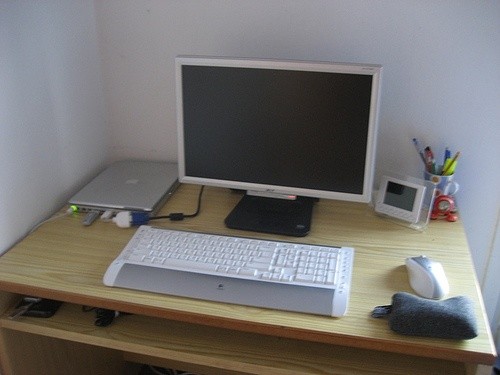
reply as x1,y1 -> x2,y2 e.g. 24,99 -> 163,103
425,170 -> 459,210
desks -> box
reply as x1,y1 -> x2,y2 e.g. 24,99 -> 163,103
0,184 -> 498,375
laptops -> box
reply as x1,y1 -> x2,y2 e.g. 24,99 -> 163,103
68,160 -> 182,219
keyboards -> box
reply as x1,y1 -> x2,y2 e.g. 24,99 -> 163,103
103,224 -> 355,318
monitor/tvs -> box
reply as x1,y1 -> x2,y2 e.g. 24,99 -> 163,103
174,56 -> 383,204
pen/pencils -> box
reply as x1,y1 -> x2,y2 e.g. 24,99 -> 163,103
413,139 -> 460,175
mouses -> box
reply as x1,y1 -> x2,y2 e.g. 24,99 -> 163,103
405,254 -> 450,300
